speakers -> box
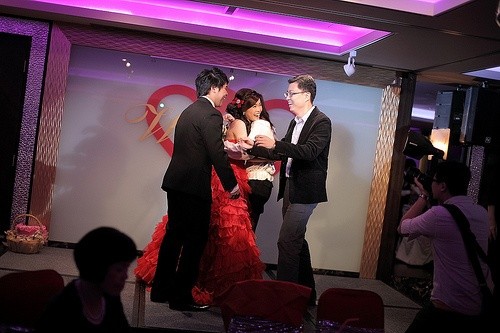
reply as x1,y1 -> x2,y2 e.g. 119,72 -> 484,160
433,85 -> 500,148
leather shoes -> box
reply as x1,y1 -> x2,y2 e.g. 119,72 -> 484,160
169,302 -> 212,311
150,293 -> 171,303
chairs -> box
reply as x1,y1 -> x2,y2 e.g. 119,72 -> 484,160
316,289 -> 385,333
218,279 -> 312,333
0,269 -> 65,328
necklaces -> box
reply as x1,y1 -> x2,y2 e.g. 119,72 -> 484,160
76,282 -> 106,324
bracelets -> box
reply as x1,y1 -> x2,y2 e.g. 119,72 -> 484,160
418,193 -> 428,201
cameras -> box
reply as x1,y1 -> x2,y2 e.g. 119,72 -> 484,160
403,128 -> 445,194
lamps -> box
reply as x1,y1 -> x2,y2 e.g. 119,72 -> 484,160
343,50 -> 357,77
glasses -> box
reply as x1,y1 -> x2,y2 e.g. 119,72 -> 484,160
283,91 -> 309,98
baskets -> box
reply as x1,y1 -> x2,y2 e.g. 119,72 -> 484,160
7,214 -> 44,254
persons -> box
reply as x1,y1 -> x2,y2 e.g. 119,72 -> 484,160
397,158 -> 496,333
239,74 -> 332,306
150,67 -> 239,312
190,88 -> 277,302
42,227 -> 137,333
